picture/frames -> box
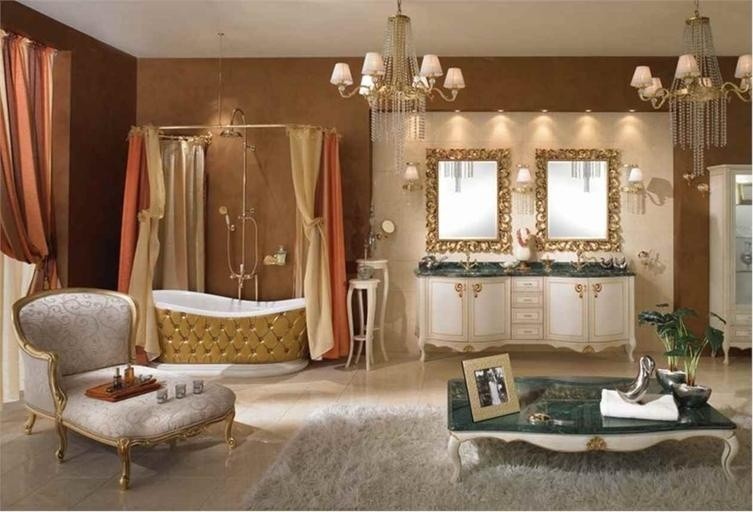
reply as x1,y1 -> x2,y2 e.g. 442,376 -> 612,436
458,351 -> 522,423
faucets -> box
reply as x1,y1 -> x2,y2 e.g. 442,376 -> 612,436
462,248 -> 472,270
576,249 -> 584,270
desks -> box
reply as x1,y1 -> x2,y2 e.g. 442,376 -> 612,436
343,278 -> 380,370
444,374 -> 742,486
353,258 -> 390,363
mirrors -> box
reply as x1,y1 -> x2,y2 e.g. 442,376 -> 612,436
422,146 -> 513,255
532,145 -> 624,253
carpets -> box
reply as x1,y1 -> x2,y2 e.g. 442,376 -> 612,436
238,400 -> 750,509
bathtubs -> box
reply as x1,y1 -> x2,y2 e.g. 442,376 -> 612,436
150,290 -> 310,380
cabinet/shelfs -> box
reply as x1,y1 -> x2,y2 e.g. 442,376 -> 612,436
414,261 -> 638,365
704,163 -> 750,365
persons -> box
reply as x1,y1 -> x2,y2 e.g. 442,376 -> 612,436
486,371 -> 500,406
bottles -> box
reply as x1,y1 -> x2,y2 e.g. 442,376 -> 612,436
113,367 -> 122,388
125,363 -> 135,385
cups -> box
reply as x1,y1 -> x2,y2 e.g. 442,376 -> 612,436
192,379 -> 204,394
158,389 -> 168,404
175,384 -> 186,399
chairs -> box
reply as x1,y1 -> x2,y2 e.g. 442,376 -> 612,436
9,287 -> 238,492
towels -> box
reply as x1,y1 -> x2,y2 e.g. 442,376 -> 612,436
598,387 -> 680,423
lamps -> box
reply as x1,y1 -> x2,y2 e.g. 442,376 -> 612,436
622,164 -> 644,193
628,1 -> 751,180
513,164 -> 534,194
327,1 -> 468,180
398,160 -> 423,192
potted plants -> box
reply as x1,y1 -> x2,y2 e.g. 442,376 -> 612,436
670,308 -> 726,409
633,298 -> 698,392
